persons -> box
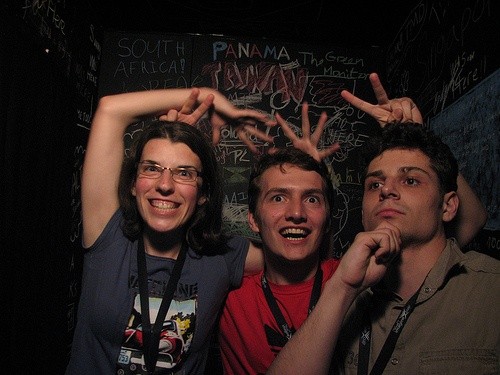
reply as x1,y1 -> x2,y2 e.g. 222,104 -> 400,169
158,88 -> 486,375
64,86 -> 338,375
261,122 -> 500,375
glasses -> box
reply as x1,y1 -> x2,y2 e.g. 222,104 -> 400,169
138,161 -> 202,184
